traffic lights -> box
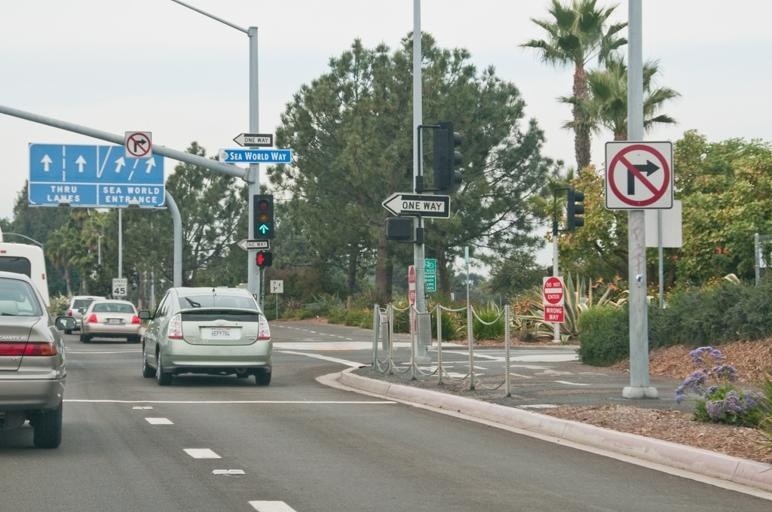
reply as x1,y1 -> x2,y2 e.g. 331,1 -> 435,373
252,192 -> 275,268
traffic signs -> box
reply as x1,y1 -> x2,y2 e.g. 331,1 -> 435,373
219,148 -> 292,164
380,192 -> 451,219
28,143 -> 166,209
232,133 -> 274,148
237,238 -> 272,252
543,276 -> 565,323
425,259 -> 436,292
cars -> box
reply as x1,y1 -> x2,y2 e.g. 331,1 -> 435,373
0,237 -> 71,452
58,293 -> 143,344
135,283 -> 276,384
